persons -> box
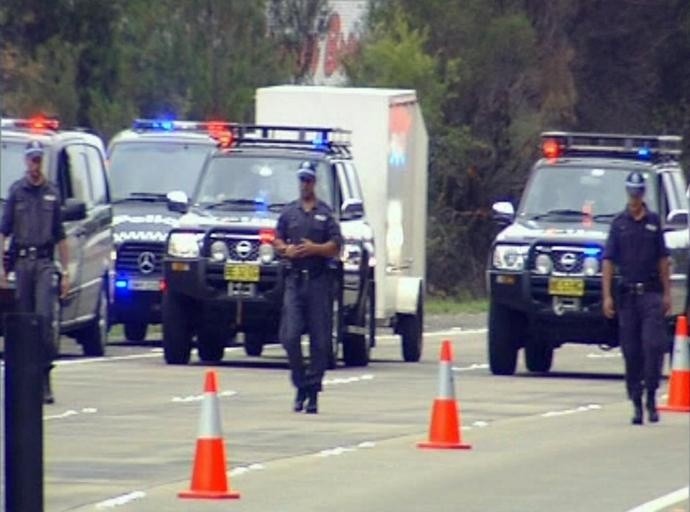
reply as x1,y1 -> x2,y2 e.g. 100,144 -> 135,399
602,170 -> 673,424
0,140 -> 70,404
274,162 -> 343,413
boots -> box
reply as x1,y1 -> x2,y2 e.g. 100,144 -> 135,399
633,391 -> 658,423
295,391 -> 317,412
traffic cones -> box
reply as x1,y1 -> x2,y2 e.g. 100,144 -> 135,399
417,338 -> 473,451
653,315 -> 690,413
176,372 -> 243,502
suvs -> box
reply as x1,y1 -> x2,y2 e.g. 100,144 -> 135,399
1,116 -> 117,354
484,129 -> 690,377
160,122 -> 379,370
101,118 -> 247,346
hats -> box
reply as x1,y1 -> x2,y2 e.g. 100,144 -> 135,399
25,140 -> 44,159
298,160 -> 315,180
625,171 -> 645,195
